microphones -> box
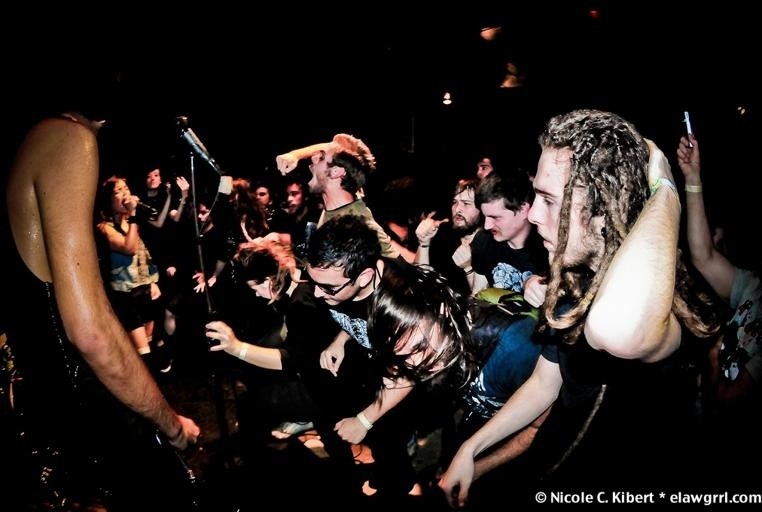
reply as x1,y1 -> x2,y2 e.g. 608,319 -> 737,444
137,202 -> 157,212
174,115 -> 226,176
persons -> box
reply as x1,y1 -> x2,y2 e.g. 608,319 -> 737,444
98,109 -> 759,512
0,62 -> 202,512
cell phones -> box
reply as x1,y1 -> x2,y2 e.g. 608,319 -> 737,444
683,111 -> 694,149
